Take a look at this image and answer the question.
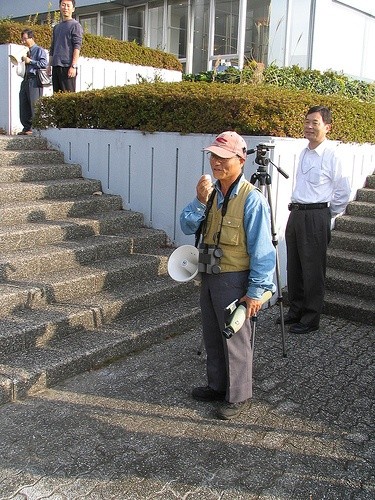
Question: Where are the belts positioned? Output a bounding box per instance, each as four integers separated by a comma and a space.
290, 203, 327, 210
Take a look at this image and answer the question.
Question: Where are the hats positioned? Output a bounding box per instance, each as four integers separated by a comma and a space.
17, 60, 26, 80
201, 131, 247, 160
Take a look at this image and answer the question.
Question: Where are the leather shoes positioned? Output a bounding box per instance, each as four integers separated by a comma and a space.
276, 315, 300, 323
289, 322, 319, 334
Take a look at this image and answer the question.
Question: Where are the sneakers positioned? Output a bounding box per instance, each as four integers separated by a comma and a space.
218, 399, 249, 419
192, 385, 227, 401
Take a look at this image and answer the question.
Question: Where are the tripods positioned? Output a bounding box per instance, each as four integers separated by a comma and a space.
245, 156, 289, 360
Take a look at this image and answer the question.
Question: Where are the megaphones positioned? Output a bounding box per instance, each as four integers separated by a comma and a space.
167, 244, 202, 287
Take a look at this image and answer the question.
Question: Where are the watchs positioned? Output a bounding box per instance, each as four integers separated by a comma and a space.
72, 64, 77, 69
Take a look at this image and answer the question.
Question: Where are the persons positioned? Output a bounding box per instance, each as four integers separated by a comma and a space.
180, 131, 276, 419
46, 0, 83, 129
10, 29, 51, 135
276, 108, 351, 334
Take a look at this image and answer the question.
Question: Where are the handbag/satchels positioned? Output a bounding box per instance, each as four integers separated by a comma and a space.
36, 47, 53, 87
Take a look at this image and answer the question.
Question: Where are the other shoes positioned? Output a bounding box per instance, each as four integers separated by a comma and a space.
18, 129, 33, 135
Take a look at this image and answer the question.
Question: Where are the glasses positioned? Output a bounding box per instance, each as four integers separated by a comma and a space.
206, 151, 219, 160
